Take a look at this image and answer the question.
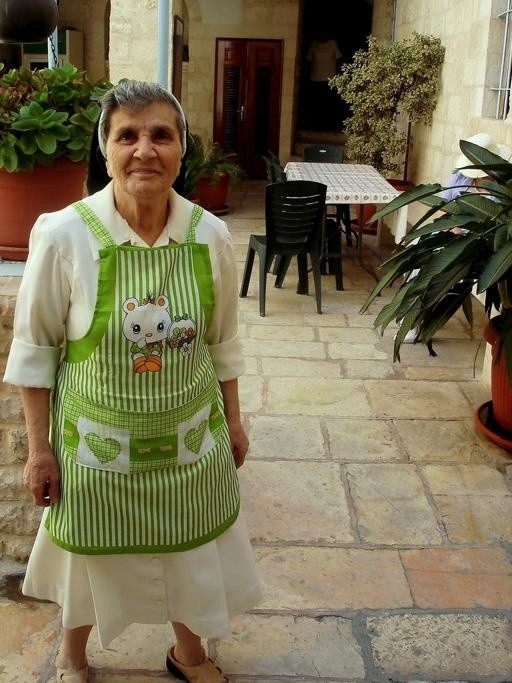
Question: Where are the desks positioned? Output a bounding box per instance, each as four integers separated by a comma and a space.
282, 161, 398, 294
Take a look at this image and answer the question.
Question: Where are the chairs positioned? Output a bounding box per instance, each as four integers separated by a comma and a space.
263, 145, 354, 292
239, 179, 333, 316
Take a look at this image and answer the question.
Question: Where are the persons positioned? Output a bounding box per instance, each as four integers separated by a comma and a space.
2, 77, 250, 682
390, 132, 511, 347
306, 34, 343, 83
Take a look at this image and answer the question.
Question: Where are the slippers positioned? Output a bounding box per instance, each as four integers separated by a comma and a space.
57, 664, 88, 683
166, 645, 229, 683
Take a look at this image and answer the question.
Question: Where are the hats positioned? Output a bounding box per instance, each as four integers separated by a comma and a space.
457, 133, 512, 178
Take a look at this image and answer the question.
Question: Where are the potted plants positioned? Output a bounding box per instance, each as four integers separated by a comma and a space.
0, 54, 127, 262
188, 135, 244, 218
366, 138, 512, 439
328, 29, 446, 236
